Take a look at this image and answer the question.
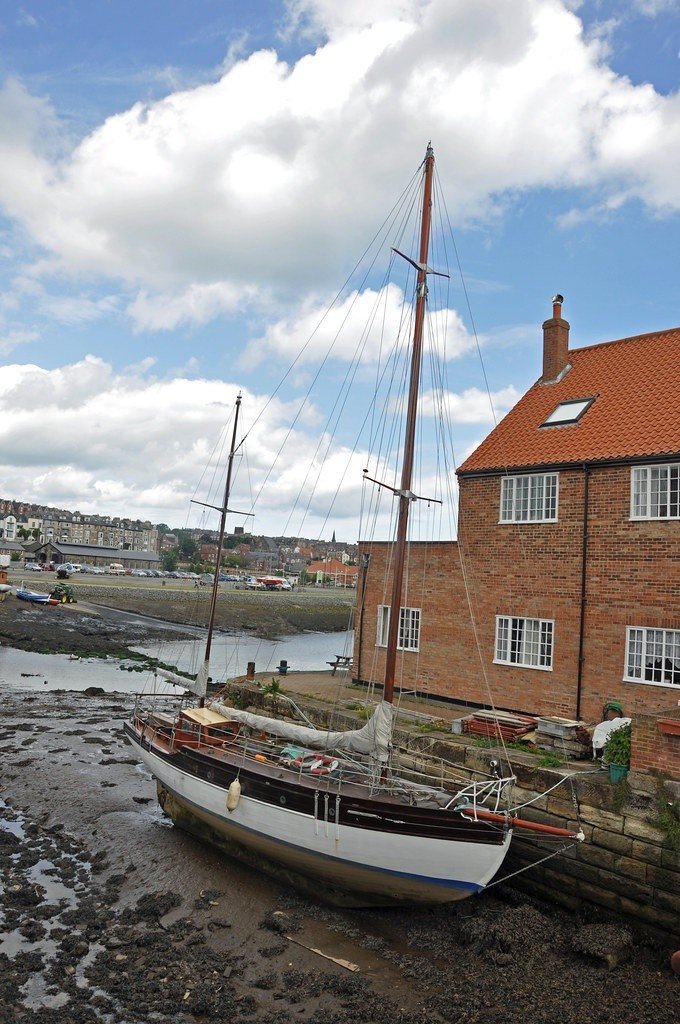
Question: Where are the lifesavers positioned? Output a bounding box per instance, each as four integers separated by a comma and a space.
310, 758, 339, 775
294, 752, 324, 767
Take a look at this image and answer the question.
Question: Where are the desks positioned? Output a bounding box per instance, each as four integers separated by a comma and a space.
327, 654, 354, 676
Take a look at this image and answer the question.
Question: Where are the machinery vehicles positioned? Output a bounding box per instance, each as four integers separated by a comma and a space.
50, 582, 77, 604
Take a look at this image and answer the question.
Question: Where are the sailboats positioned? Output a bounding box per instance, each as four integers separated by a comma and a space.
119, 140, 585, 903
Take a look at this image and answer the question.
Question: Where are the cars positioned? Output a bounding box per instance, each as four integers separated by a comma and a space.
23, 558, 292, 590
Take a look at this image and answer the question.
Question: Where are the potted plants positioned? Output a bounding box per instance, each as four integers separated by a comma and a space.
604, 722, 632, 787
